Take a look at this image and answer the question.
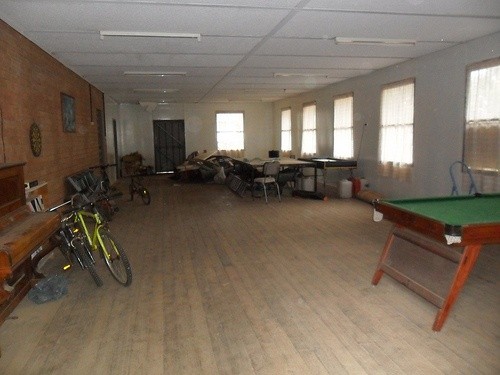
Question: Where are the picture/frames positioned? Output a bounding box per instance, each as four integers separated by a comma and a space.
60, 92, 77, 133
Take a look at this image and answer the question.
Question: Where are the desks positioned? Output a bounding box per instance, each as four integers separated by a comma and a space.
373, 193, 500, 331
236, 157, 316, 201
299, 158, 358, 201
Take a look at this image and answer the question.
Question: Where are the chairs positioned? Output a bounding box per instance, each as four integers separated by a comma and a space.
254, 161, 281, 202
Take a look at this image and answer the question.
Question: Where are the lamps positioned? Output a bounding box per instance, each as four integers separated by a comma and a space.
124, 72, 186, 75
100, 30, 203, 41
335, 37, 416, 47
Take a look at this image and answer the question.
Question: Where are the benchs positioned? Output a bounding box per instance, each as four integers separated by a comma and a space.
226, 173, 251, 198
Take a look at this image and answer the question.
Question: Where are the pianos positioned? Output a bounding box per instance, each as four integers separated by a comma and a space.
0, 162, 63, 329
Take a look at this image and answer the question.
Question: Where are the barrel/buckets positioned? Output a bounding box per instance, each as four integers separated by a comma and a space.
360, 177, 368, 191
348, 177, 360, 193
338, 179, 352, 199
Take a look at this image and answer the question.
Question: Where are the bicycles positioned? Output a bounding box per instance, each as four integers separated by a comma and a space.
128, 173, 151, 205
80, 162, 119, 221
49, 192, 133, 288
